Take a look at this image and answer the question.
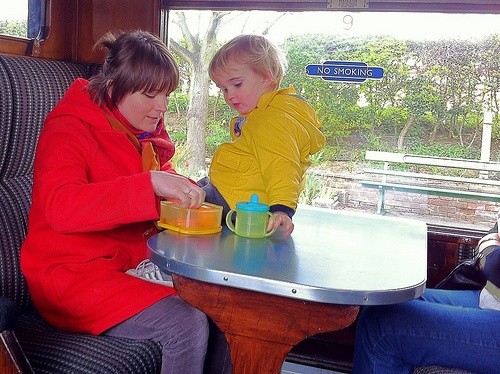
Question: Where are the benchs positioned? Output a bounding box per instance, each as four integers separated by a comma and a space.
360, 149, 500, 218
0, 53, 225, 374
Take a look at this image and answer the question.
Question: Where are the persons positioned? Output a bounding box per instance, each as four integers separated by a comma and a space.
351, 209, 500, 374
19, 29, 232, 374
196, 34, 325, 237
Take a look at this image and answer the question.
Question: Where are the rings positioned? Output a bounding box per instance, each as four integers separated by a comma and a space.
187, 187, 193, 196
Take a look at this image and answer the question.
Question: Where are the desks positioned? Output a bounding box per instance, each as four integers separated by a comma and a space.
147, 204, 427, 374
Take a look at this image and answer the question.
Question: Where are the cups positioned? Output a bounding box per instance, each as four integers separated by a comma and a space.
226, 194, 277, 239
